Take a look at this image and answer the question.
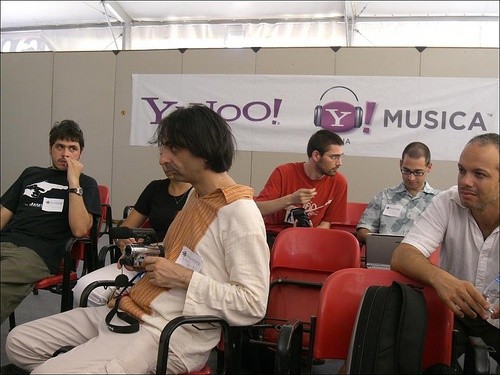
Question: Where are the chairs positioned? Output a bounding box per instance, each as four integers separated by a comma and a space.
80, 197, 453, 375
9, 184, 112, 330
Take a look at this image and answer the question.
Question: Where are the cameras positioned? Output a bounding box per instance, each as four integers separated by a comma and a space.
121, 244, 165, 267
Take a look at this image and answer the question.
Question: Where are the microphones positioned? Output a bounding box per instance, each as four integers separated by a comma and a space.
108, 226, 157, 240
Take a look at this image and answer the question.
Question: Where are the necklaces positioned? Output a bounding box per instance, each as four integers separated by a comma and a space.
172, 184, 188, 204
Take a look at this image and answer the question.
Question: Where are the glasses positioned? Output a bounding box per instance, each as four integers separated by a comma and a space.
401, 165, 427, 176
324, 154, 344, 161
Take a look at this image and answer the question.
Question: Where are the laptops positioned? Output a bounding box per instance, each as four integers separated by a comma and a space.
366, 234, 406, 267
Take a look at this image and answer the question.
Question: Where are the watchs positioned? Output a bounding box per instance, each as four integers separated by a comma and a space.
68, 187, 83, 196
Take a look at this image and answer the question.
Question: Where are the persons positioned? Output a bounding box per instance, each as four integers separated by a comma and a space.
356, 142, 439, 241
0, 120, 101, 327
5, 104, 270, 375
390, 132, 499, 375
70, 143, 194, 308
254, 130, 348, 230
